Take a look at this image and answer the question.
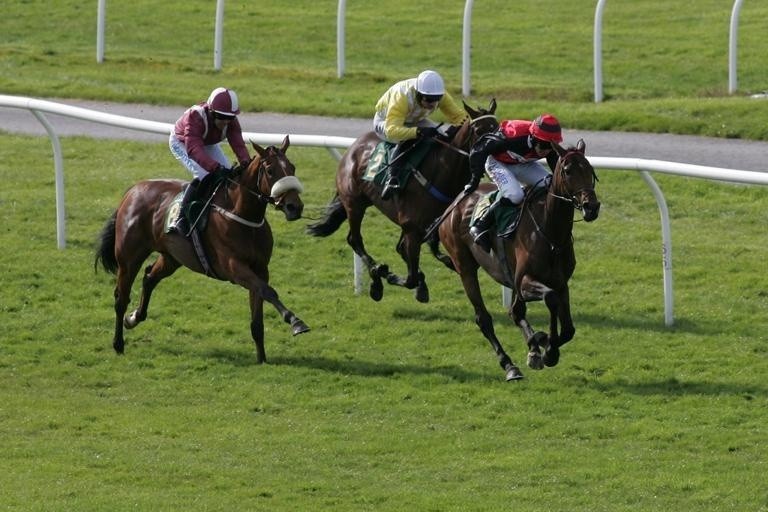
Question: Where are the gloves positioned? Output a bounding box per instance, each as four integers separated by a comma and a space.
217, 164, 228, 174
417, 127, 439, 138
463, 177, 478, 194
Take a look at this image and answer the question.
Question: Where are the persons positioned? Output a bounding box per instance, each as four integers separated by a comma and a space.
373, 70, 472, 143
169, 87, 251, 182
484, 114, 563, 209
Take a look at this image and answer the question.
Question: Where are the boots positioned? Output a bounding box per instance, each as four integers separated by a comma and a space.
163, 184, 195, 235
380, 147, 402, 201
469, 198, 519, 253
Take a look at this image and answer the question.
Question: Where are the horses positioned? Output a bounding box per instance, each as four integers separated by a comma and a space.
426, 138, 601, 382
306, 99, 499, 303
94, 134, 311, 365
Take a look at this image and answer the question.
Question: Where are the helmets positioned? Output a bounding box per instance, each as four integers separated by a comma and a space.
415, 70, 445, 95
207, 87, 241, 118
529, 115, 563, 144
